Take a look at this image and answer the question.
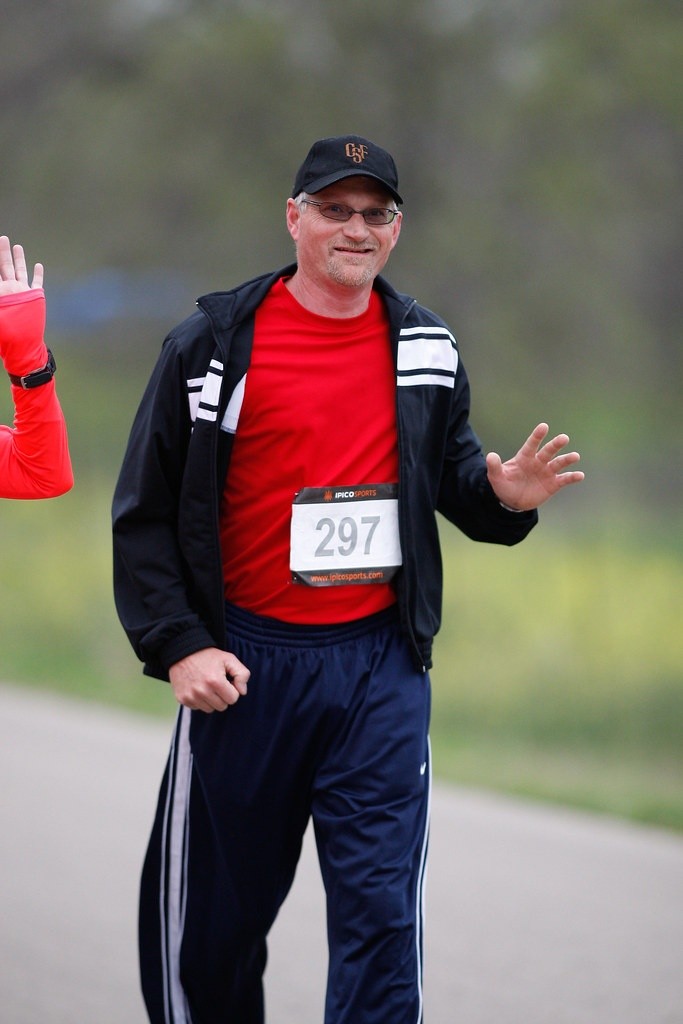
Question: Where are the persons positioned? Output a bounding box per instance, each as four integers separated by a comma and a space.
0, 236, 75, 504
113, 138, 585, 1024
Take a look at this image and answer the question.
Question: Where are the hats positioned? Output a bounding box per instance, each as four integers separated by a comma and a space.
292, 134, 403, 206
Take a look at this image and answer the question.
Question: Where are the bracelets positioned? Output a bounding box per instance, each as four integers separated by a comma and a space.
7, 347, 58, 388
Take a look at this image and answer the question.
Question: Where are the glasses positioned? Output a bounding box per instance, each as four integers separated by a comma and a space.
301, 198, 399, 225
